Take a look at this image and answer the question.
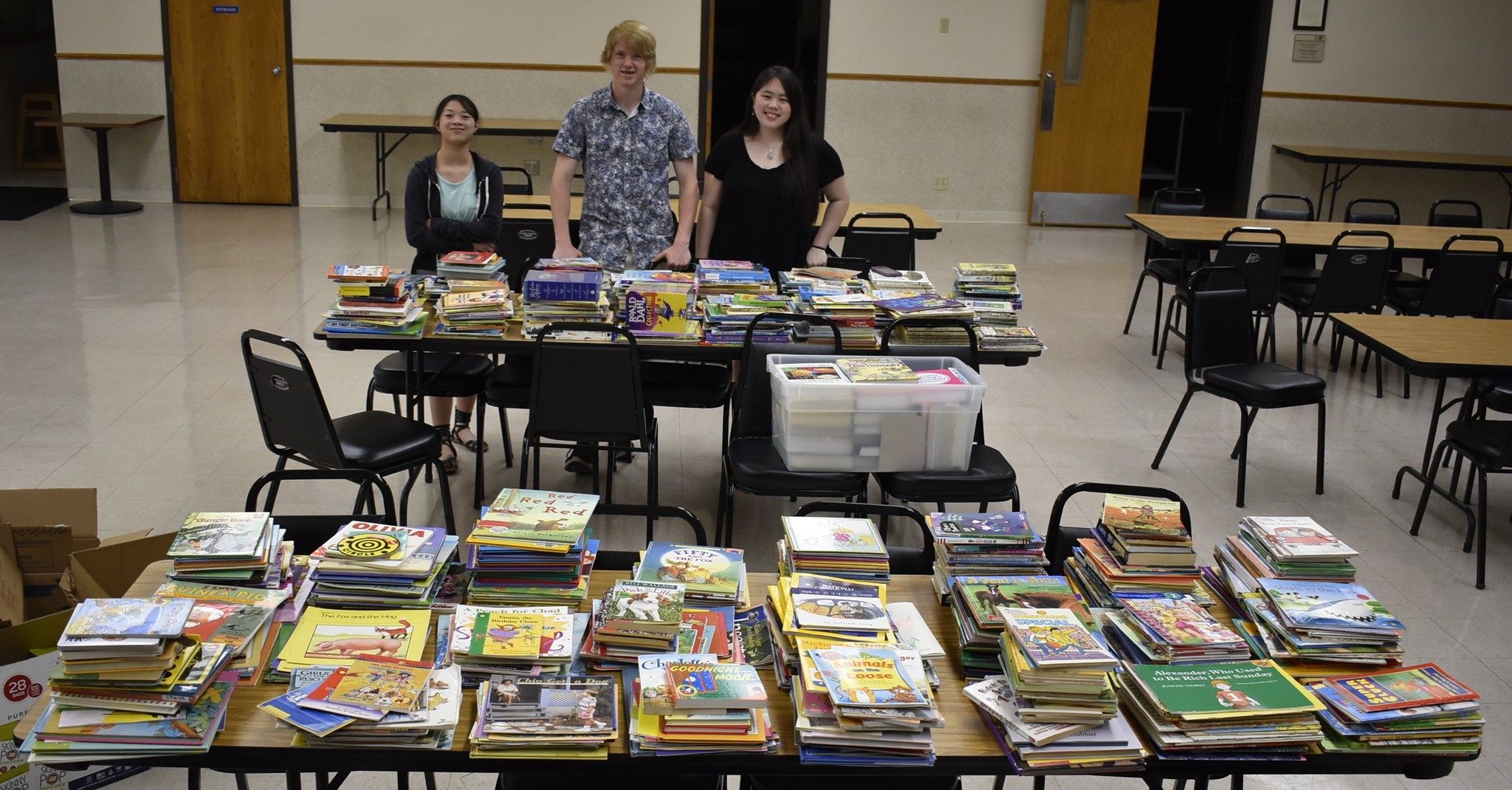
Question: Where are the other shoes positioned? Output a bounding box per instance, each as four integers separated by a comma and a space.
573, 728, 590, 732
598, 723, 607, 729
617, 442, 634, 464
564, 442, 596, 474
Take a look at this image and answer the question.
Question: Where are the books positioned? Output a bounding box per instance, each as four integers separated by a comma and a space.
778, 359, 975, 475
321, 251, 1041, 354
18, 490, 1486, 769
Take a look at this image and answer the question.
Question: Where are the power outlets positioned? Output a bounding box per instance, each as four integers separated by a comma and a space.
524, 161, 540, 176
527, 137, 543, 145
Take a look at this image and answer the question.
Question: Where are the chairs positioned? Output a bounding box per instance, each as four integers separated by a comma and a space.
187, 167, 1238, 790
1122, 194, 1512, 590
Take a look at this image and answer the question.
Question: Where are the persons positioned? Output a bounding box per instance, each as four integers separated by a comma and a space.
574, 689, 607, 732
497, 679, 518, 706
549, 20, 695, 473
405, 94, 504, 471
691, 66, 850, 388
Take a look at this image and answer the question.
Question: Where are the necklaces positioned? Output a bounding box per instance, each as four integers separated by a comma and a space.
756, 138, 783, 160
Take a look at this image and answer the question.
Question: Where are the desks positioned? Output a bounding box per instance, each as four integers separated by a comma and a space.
1272, 144, 1512, 223
502, 194, 943, 240
34, 112, 165, 215
14, 563, 1480, 790
312, 294, 1043, 366
320, 113, 564, 222
1125, 213, 1512, 318
1330, 313, 1512, 552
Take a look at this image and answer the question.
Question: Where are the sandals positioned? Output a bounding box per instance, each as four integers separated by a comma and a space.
436, 424, 458, 471
450, 406, 489, 453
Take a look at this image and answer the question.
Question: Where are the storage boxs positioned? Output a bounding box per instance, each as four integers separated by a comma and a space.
0, 488, 181, 790
766, 353, 987, 472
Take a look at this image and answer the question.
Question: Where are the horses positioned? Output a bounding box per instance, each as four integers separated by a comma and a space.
975, 584, 1010, 616
1011, 593, 1091, 623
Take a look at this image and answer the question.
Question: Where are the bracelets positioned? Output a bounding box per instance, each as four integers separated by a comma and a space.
812, 245, 825, 252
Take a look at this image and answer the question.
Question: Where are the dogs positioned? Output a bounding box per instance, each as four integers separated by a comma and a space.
614, 591, 671, 621
